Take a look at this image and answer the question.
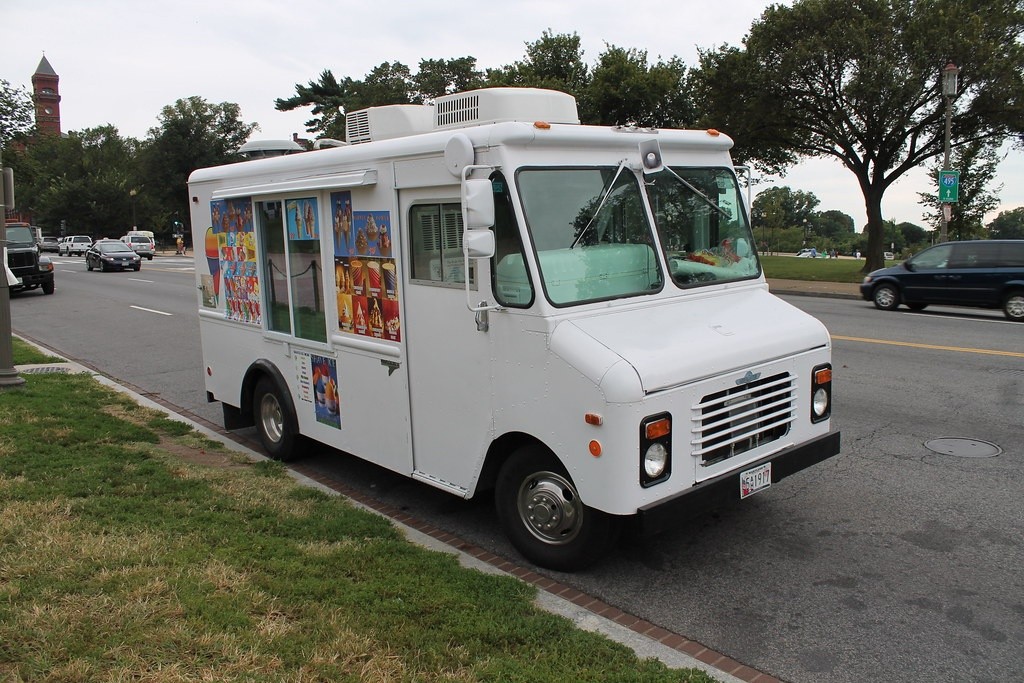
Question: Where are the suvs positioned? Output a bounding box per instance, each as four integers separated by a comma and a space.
37, 237, 60, 253
57, 236, 92, 257
4, 222, 54, 298
120, 235, 155, 260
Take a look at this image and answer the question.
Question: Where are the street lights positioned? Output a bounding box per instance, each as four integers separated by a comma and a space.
130, 188, 137, 231
940, 60, 957, 241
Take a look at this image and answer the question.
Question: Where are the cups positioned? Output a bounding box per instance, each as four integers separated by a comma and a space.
351, 260, 363, 296
316, 374, 337, 415
367, 261, 381, 298
381, 263, 396, 299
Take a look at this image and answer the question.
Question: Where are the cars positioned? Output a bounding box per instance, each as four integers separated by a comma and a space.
85, 237, 126, 257
86, 244, 141, 273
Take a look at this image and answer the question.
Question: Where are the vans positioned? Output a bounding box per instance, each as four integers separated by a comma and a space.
128, 231, 156, 247
859, 240, 1024, 322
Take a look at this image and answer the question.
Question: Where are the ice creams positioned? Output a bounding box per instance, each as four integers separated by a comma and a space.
212, 201, 253, 232
355, 214, 391, 257
313, 363, 338, 416
333, 199, 351, 250
295, 200, 315, 238
336, 269, 399, 340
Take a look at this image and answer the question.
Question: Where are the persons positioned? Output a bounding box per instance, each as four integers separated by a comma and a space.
811, 247, 816, 258
177, 236, 186, 254
822, 249, 838, 259
856, 250, 861, 259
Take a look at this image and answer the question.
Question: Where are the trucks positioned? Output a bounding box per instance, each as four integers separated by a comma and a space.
186, 85, 841, 572
